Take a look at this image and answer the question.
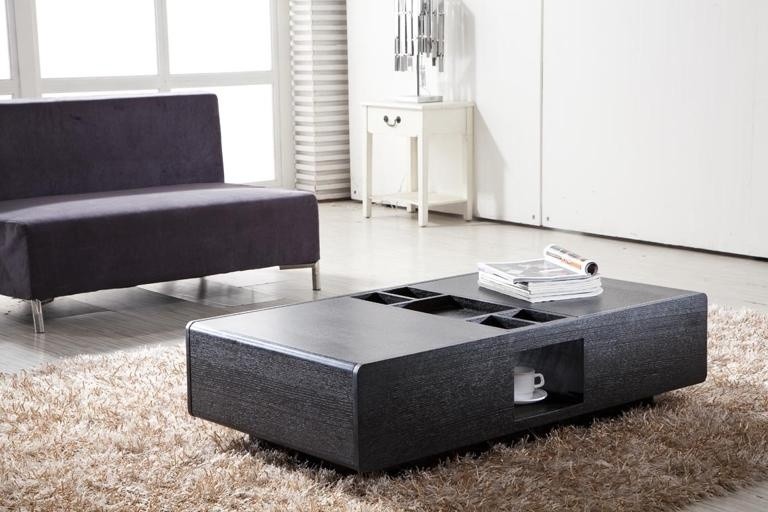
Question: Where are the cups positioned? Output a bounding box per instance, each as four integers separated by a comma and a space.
514, 366, 545, 396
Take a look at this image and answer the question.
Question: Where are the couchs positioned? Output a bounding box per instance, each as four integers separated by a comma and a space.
0, 94, 320, 333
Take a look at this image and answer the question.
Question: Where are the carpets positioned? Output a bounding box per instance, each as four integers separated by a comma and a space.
0, 305, 768, 512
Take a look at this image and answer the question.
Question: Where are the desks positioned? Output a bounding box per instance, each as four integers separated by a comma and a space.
185, 272, 708, 473
363, 101, 476, 227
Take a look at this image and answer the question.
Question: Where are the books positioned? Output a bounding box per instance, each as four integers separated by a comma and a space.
476, 242, 604, 303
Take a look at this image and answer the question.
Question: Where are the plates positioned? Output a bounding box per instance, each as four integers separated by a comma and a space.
514, 389, 548, 405
